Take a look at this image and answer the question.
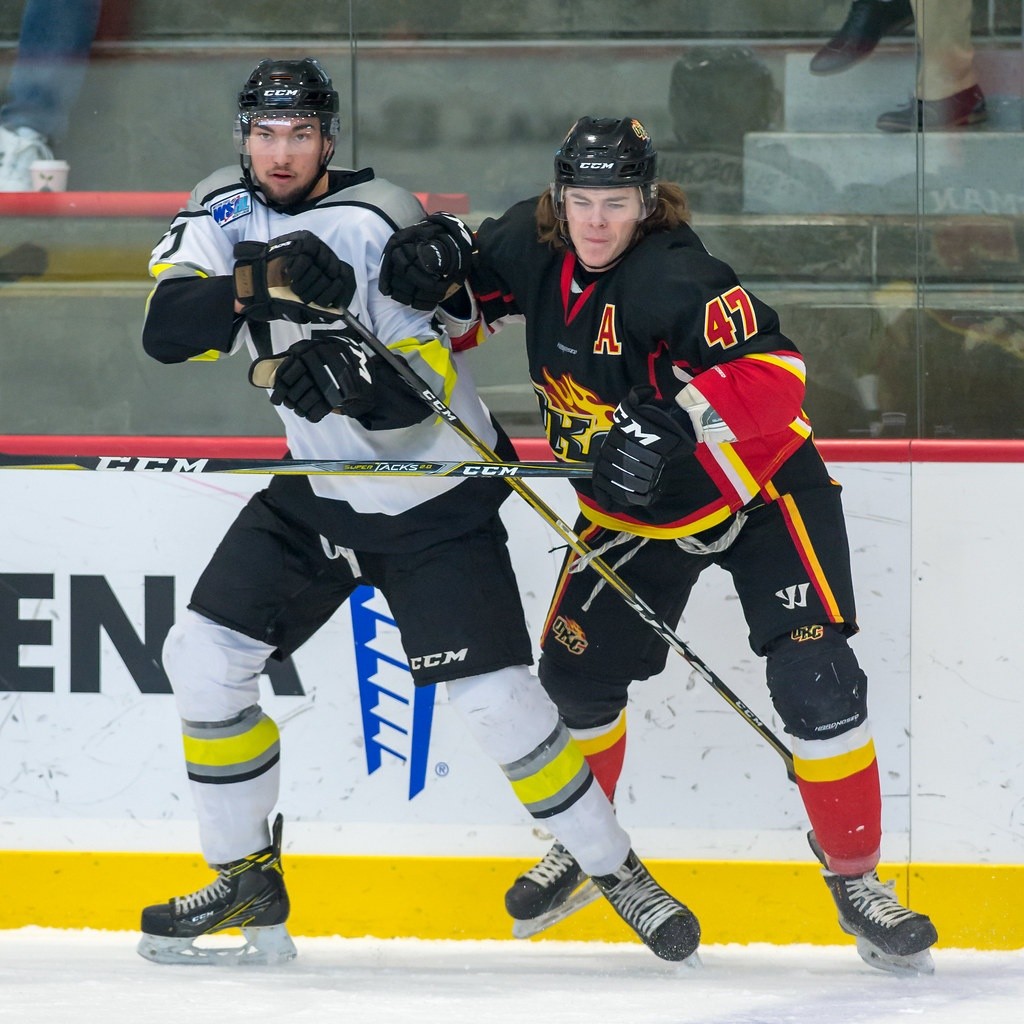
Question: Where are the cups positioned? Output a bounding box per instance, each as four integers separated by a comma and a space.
30, 159, 70, 192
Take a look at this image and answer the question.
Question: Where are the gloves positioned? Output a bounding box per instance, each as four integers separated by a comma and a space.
592, 385, 698, 513
249, 336, 380, 424
378, 211, 478, 312
232, 230, 357, 325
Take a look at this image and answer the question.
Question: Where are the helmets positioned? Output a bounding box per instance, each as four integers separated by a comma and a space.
555, 116, 657, 218
668, 45, 774, 143
238, 58, 340, 139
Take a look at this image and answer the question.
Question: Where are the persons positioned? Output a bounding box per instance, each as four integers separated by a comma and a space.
0, 0, 103, 193
140, 56, 701, 963
378, 117, 938, 957
651, 45, 1024, 440
810, 0, 987, 133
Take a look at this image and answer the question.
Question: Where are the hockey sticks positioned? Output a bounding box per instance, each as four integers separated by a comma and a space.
341, 308, 800, 787
1, 453, 600, 479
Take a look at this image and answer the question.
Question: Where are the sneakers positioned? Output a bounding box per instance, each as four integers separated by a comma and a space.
503, 783, 617, 939
136, 813, 298, 969
807, 829, 938, 977
0, 126, 53, 192
590, 848, 704, 972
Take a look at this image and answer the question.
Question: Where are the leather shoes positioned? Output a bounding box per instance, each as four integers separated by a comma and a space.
809, 0, 916, 76
875, 82, 987, 132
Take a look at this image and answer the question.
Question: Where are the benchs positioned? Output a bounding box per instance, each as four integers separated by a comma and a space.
1, 1, 1024, 471
